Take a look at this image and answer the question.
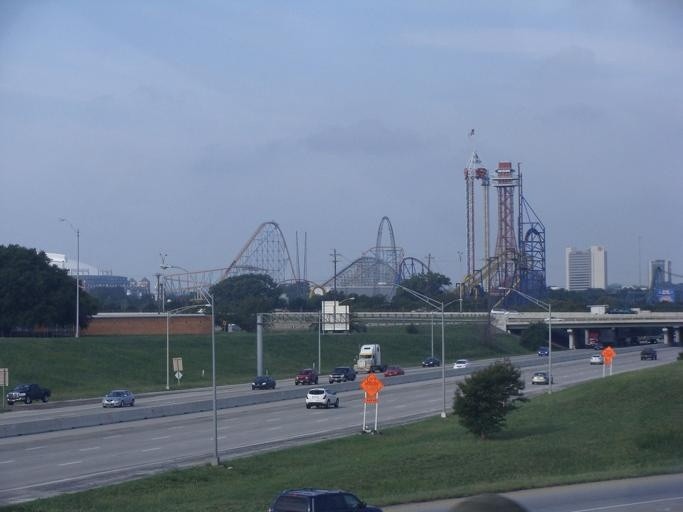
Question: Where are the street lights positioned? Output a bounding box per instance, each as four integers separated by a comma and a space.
498, 286, 552, 395
318, 297, 356, 374
378, 281, 464, 418
59, 216, 80, 337
159, 250, 220, 466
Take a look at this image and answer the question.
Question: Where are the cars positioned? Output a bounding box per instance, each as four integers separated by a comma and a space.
453, 359, 469, 369
590, 355, 603, 365
269, 487, 382, 512
102, 389, 136, 408
605, 307, 638, 314
306, 388, 339, 409
295, 368, 318, 385
640, 348, 657, 360
538, 347, 549, 356
532, 372, 553, 385
251, 376, 276, 390
329, 367, 356, 384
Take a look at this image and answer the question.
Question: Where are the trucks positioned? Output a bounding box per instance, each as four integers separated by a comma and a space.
353, 343, 387, 373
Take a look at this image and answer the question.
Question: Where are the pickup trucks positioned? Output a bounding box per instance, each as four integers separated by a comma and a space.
6, 383, 51, 405
